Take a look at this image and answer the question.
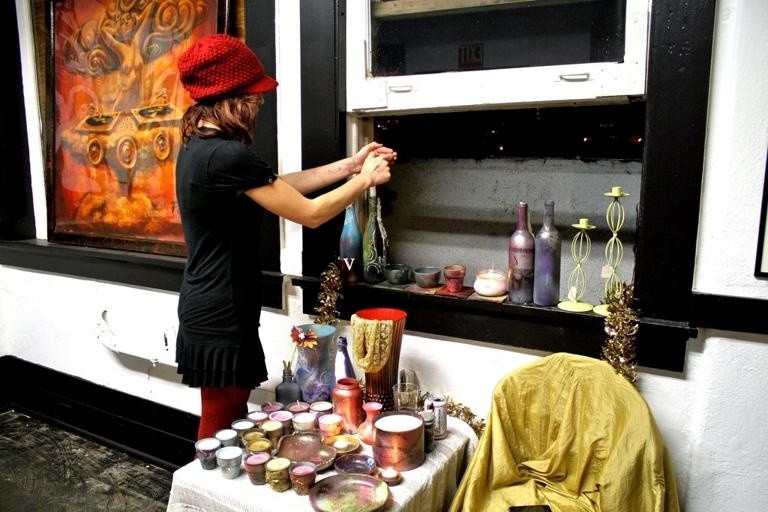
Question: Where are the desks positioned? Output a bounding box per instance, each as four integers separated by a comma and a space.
163, 416, 478, 512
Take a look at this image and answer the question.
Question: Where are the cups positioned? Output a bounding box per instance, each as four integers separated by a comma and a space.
195, 439, 220, 469
310, 402, 332, 426
444, 264, 466, 294
265, 458, 291, 493
260, 421, 284, 444
215, 428, 238, 446
393, 383, 417, 415
269, 410, 293, 433
244, 452, 271, 485
371, 411, 425, 472
247, 438, 272, 454
318, 414, 343, 436
232, 419, 255, 436
293, 412, 314, 432
247, 411, 268, 425
242, 429, 265, 445
216, 446, 241, 479
261, 401, 285, 412
288, 401, 309, 412
289, 462, 316, 497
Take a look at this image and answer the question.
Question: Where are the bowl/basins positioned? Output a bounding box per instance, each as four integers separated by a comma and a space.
413, 266, 441, 288
271, 429, 338, 471
335, 454, 377, 473
473, 270, 507, 296
323, 435, 361, 453
385, 263, 412, 285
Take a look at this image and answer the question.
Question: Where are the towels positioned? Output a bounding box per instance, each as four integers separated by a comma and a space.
351, 314, 392, 374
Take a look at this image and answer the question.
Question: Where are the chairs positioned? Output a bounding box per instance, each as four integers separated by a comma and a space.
451, 353, 680, 512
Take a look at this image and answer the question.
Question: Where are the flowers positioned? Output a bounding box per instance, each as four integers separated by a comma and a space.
291, 326, 317, 348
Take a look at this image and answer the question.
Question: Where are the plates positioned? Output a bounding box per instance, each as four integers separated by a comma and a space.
310, 474, 389, 512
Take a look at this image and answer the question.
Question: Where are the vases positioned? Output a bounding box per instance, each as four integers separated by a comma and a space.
355, 307, 408, 410
296, 323, 337, 402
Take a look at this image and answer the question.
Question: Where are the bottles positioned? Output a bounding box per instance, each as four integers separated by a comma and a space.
274, 369, 301, 403
340, 202, 363, 284
332, 378, 362, 429
421, 412, 437, 453
534, 200, 561, 307
362, 195, 387, 283
509, 202, 534, 303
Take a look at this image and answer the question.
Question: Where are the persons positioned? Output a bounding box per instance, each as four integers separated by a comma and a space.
174, 33, 398, 441
99, 1, 158, 113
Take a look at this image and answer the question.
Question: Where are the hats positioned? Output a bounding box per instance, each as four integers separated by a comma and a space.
177, 33, 279, 102
